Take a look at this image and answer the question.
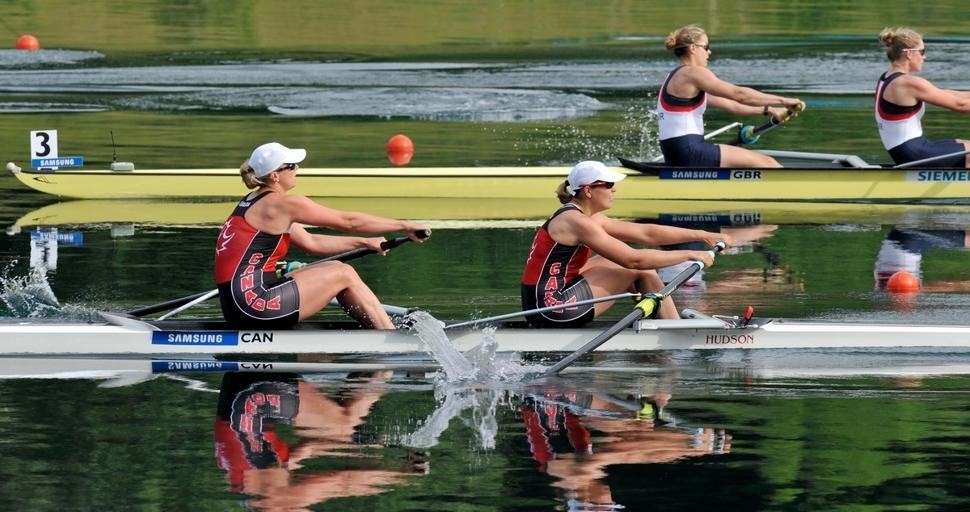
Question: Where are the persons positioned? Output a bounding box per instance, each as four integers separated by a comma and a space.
658, 26, 806, 168
214, 368, 429, 508
664, 216, 806, 312
216, 143, 432, 329
526, 351, 735, 511
872, 227, 970, 294
876, 26, 970, 169
517, 159, 734, 316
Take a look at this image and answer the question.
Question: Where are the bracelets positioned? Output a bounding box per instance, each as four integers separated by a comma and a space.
762, 104, 773, 115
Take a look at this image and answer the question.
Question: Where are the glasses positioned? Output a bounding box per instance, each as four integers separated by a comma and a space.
695, 43, 710, 52
581, 182, 615, 190
276, 164, 297, 172
902, 47, 927, 56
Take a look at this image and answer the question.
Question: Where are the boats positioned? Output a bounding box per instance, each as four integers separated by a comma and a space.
2, 351, 970, 395
3, 155, 970, 201
2, 308, 970, 358
11, 194, 967, 240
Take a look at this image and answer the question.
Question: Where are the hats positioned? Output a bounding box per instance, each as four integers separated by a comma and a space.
247, 142, 308, 179
566, 160, 627, 190
